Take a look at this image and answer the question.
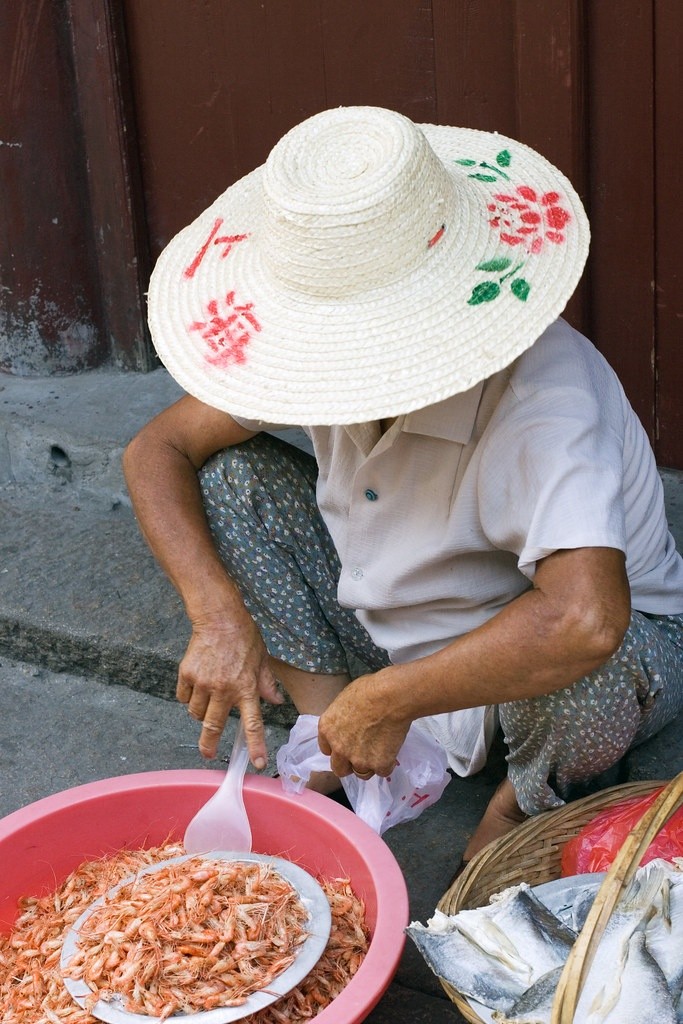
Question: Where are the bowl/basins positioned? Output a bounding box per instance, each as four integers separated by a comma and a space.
0, 767, 409, 1024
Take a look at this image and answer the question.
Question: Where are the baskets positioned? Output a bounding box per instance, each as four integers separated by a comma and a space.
429, 771, 683, 1024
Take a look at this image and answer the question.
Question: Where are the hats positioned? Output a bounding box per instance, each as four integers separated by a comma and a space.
146, 104, 590, 426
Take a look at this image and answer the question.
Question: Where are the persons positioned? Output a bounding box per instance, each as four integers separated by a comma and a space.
122, 105, 683, 892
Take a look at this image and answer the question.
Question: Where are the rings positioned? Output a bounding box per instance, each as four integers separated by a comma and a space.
353, 769, 372, 776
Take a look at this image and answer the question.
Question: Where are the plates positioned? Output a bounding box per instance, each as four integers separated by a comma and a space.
60, 851, 332, 1024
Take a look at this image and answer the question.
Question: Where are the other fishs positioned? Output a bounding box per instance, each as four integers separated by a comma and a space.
403, 856, 683, 1024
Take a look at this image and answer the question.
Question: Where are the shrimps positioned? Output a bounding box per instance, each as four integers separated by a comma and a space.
0, 828, 369, 1024
